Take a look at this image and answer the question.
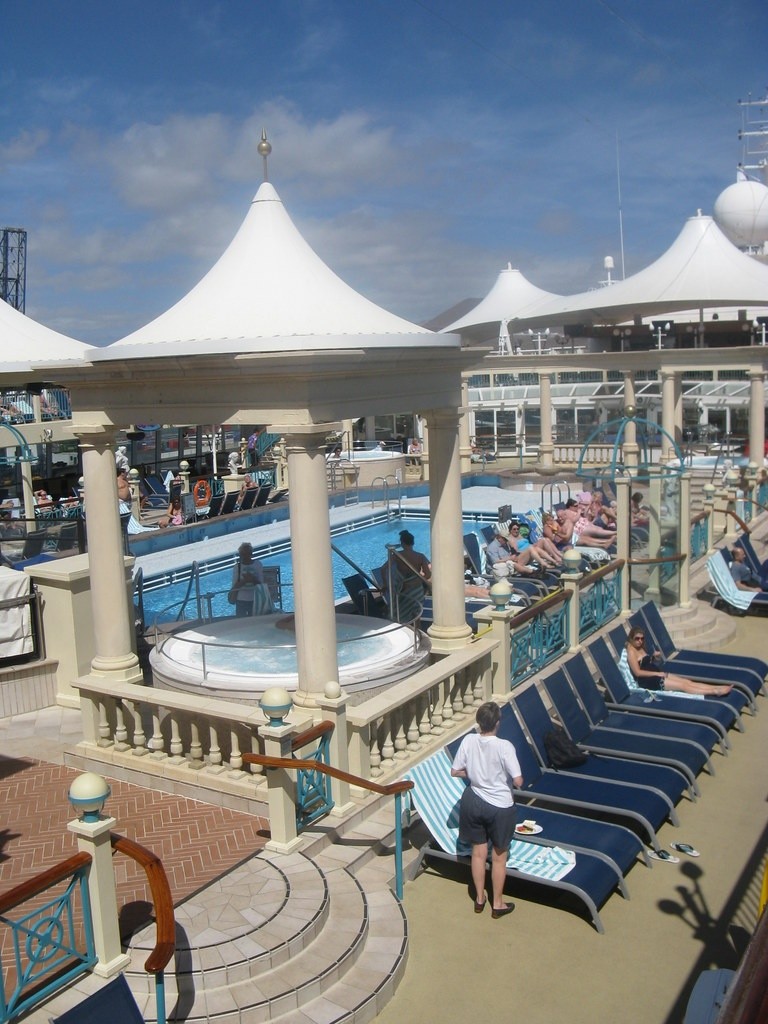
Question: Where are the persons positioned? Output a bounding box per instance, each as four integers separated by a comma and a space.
116, 446, 130, 473
34, 490, 79, 513
0, 500, 25, 528
486, 528, 561, 577
471, 441, 489, 458
564, 490, 617, 549
507, 522, 563, 562
140, 491, 148, 509
166, 499, 182, 525
232, 543, 264, 617
334, 447, 341, 457
410, 438, 422, 465
0, 391, 59, 417
731, 547, 768, 592
542, 509, 617, 548
117, 467, 132, 509
248, 430, 261, 466
624, 626, 734, 698
228, 452, 240, 474
503, 541, 562, 569
238, 475, 259, 504
380, 530, 514, 629
631, 492, 674, 526
450, 702, 523, 919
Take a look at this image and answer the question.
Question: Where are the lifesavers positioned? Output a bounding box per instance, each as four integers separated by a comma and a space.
194, 480, 211, 506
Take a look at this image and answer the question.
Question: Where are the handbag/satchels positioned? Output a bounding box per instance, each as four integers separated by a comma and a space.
227, 589, 238, 604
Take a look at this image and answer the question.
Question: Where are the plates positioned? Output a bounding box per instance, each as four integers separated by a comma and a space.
514, 823, 544, 835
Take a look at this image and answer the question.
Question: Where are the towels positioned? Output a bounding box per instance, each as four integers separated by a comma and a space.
617, 647, 703, 700
162, 470, 175, 493
531, 508, 611, 562
576, 491, 592, 505
704, 551, 759, 611
469, 531, 488, 586
398, 746, 577, 882
251, 583, 286, 616
493, 519, 515, 535
119, 498, 160, 534
386, 569, 425, 624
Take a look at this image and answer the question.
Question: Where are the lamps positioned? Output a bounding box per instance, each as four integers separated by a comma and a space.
563, 550, 582, 574
748, 462, 758, 475
518, 404, 522, 415
68, 772, 111, 823
273, 446, 281, 456
129, 469, 138, 481
727, 473, 738, 489
180, 461, 189, 472
488, 582, 512, 612
78, 477, 84, 488
702, 484, 716, 500
258, 687, 295, 728
698, 401, 703, 413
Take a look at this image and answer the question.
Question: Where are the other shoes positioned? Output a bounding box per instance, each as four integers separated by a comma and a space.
475, 896, 487, 913
538, 565, 546, 578
492, 903, 515, 920
529, 568, 542, 580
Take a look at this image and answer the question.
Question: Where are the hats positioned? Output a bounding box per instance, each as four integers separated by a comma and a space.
498, 529, 511, 541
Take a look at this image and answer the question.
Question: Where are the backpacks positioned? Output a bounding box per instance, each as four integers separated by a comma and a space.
544, 727, 588, 773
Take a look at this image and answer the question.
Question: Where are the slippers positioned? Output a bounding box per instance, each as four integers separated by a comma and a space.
669, 842, 700, 856
648, 850, 680, 864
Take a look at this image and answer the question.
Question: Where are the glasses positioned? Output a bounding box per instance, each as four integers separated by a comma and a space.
571, 505, 579, 508
633, 636, 644, 642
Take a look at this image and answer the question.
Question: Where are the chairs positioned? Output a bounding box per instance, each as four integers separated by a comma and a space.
399, 600, 768, 935
0, 471, 289, 566
0, 390, 71, 425
705, 534, 768, 610
471, 454, 497, 464
407, 438, 419, 466
341, 482, 650, 635
